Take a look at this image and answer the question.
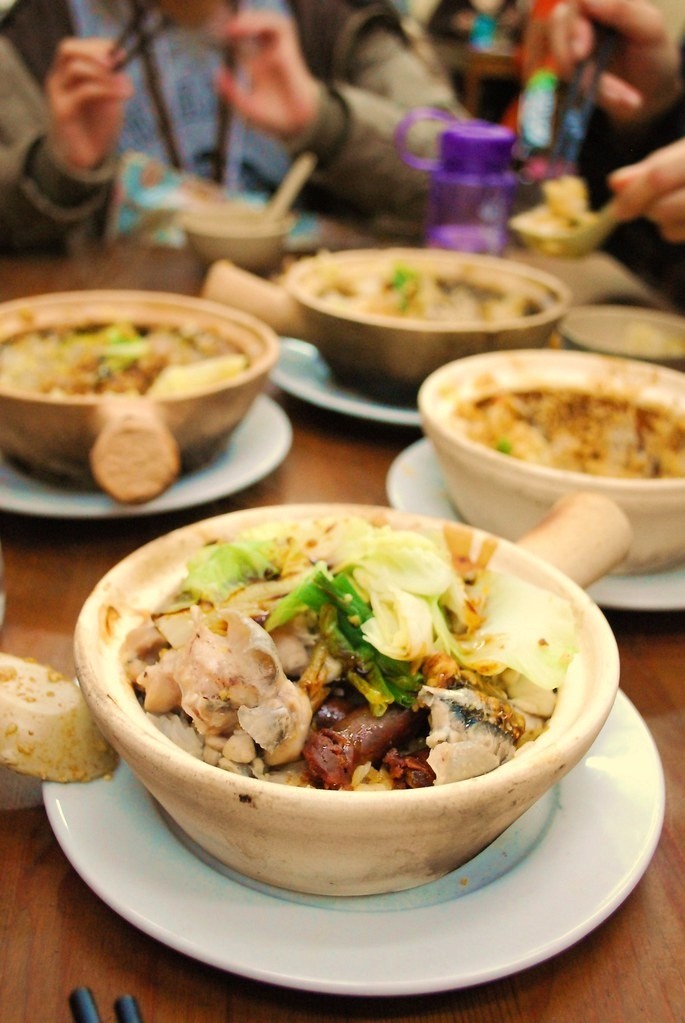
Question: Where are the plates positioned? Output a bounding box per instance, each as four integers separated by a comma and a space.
267, 335, 420, 425
0, 390, 291, 515
556, 302, 685, 362
385, 439, 685, 611
42, 672, 666, 996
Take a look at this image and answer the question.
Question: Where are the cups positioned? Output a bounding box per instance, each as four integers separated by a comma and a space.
393, 106, 513, 257
470, 16, 496, 52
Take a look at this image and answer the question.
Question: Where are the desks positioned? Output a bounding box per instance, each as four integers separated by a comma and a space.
0, 231, 684, 1023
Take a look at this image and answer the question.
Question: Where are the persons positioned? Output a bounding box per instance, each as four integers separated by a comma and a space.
427, 0, 559, 134
0, 0, 475, 251
545, 0, 685, 243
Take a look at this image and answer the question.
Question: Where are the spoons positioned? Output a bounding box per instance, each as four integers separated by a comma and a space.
507, 195, 621, 240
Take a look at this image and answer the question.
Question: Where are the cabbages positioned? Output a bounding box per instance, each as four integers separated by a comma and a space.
39, 315, 259, 401
184, 511, 577, 698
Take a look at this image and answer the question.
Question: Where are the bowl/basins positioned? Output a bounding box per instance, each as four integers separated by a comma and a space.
71, 493, 636, 890
0, 290, 280, 505
183, 211, 297, 269
204, 250, 574, 406
418, 349, 685, 574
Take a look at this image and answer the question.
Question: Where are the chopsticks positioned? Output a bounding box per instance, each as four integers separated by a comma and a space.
527, 41, 611, 180
68, 987, 143, 1023
105, 0, 167, 75
